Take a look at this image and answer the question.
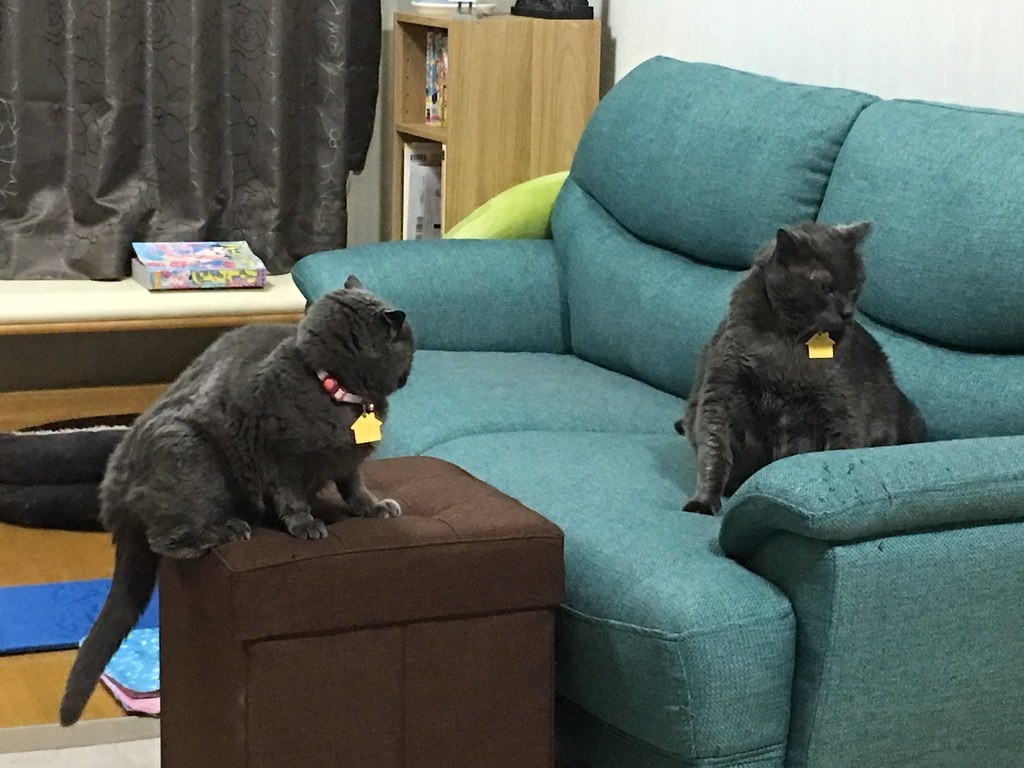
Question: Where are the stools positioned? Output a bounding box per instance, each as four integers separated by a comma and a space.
159, 456, 568, 768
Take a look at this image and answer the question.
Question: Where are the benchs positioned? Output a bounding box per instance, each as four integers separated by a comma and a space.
0, 271, 307, 393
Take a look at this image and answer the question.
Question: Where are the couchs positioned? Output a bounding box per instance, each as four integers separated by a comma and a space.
290, 55, 1024, 768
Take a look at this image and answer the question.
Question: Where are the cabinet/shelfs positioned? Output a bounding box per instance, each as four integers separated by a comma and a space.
389, 12, 602, 241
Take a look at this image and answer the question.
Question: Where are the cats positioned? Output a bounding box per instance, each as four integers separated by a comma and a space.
58, 275, 414, 728
673, 222, 926, 516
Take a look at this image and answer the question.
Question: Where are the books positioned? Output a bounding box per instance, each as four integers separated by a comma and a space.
401, 30, 448, 245
130, 239, 267, 290
